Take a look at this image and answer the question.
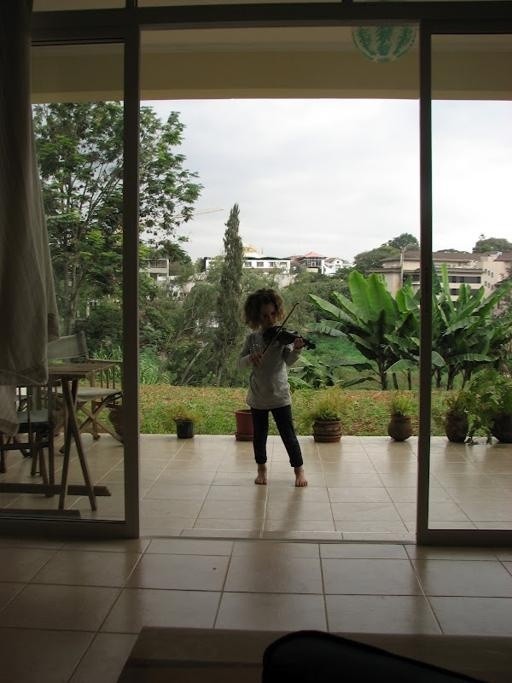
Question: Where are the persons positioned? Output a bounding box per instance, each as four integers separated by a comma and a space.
235, 287, 310, 487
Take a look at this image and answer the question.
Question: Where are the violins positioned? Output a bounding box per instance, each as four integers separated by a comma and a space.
263, 325, 316, 350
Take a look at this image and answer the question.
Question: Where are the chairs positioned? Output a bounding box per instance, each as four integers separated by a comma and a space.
0, 331, 123, 517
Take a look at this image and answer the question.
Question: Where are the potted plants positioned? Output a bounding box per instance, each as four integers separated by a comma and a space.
234, 378, 312, 441
301, 388, 353, 443
433, 368, 512, 446
165, 403, 204, 439
388, 397, 417, 441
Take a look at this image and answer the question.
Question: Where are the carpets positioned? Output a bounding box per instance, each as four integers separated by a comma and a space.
116, 627, 512, 683
